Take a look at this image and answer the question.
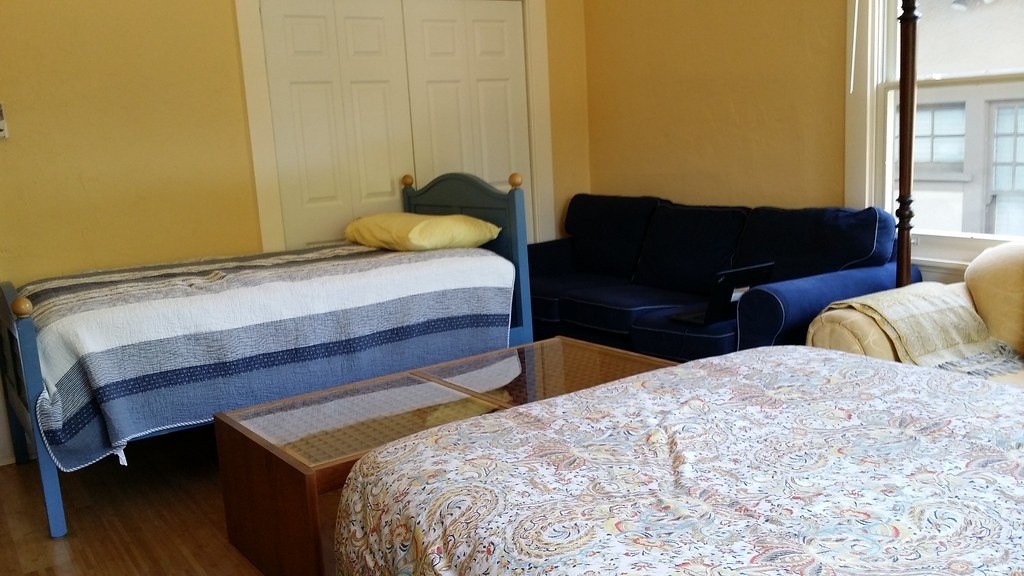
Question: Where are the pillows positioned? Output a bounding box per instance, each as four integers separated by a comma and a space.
344, 212, 503, 251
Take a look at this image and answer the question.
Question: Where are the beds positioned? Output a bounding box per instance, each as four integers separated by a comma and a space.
0, 170, 539, 539
339, 346, 1024, 576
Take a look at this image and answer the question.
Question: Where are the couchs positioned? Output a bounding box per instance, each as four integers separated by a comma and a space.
807, 235, 1024, 387
520, 186, 916, 350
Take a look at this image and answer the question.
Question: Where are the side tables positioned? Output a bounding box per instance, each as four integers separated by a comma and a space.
209, 331, 683, 573
